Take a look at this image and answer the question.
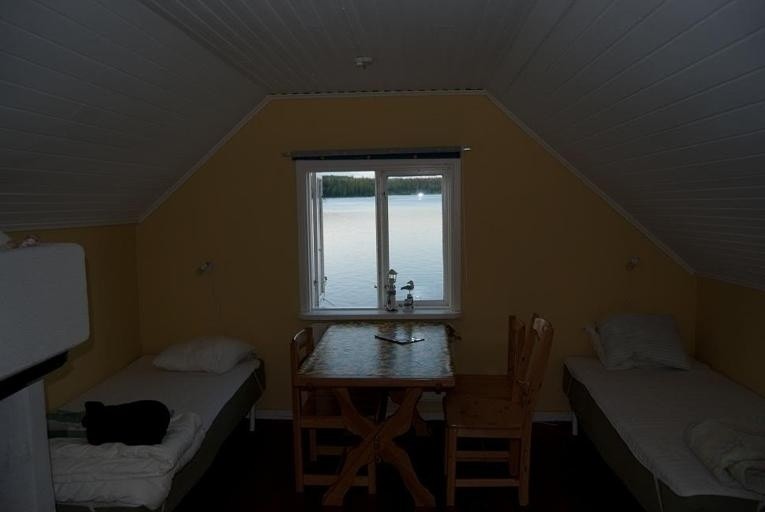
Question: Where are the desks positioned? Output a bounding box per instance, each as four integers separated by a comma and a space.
291, 320, 457, 506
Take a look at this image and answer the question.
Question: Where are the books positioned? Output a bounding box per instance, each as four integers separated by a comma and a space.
374, 329, 425, 344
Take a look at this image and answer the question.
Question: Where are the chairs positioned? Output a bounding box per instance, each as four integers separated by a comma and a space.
290, 327, 379, 495
443, 315, 529, 402
442, 316, 553, 507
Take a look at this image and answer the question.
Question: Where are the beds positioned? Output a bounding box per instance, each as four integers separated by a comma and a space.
47, 354, 265, 512
562, 356, 765, 512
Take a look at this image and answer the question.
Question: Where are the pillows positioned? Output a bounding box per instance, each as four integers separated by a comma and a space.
152, 338, 256, 375
592, 318, 689, 370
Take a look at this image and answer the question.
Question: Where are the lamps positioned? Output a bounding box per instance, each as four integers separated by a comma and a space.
625, 256, 641, 272
